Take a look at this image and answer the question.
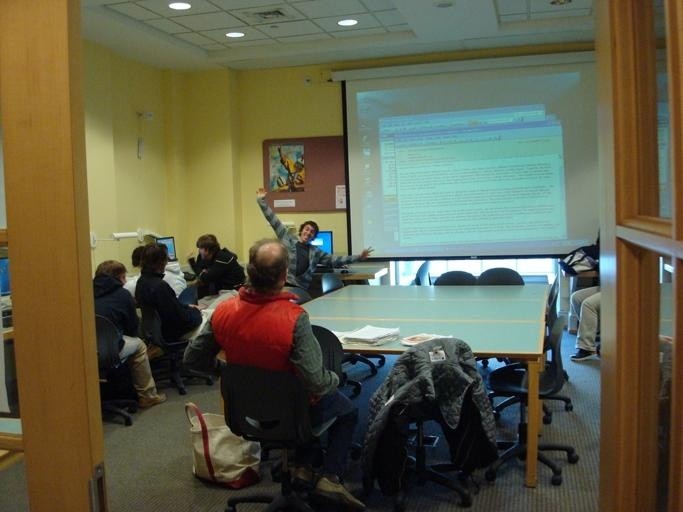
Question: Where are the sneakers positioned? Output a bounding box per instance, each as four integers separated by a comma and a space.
570, 348, 597, 361
139, 392, 167, 408
315, 478, 366, 511
290, 468, 313, 490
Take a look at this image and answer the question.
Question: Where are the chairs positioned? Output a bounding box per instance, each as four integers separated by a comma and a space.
365, 338, 473, 503
221, 369, 352, 510
92, 259, 565, 425
490, 310, 568, 427
486, 316, 567, 486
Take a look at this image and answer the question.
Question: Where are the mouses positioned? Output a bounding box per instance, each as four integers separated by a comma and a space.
341, 270, 349, 273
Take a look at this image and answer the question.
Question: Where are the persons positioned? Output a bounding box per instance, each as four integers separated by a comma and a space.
181, 237, 368, 510
134, 241, 209, 340
255, 186, 375, 289
92, 259, 169, 408
569, 284, 602, 361
176, 233, 248, 381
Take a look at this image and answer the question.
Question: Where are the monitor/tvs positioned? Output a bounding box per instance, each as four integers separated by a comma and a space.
157, 236, 177, 260
306, 230, 334, 257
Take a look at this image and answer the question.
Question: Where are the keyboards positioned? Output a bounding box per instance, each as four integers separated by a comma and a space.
315, 266, 334, 272
183, 272, 195, 281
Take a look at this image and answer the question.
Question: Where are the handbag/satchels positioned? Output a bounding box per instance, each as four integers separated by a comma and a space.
558, 245, 599, 276
185, 402, 262, 489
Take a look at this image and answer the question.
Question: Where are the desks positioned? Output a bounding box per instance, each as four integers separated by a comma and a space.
300, 283, 551, 486
